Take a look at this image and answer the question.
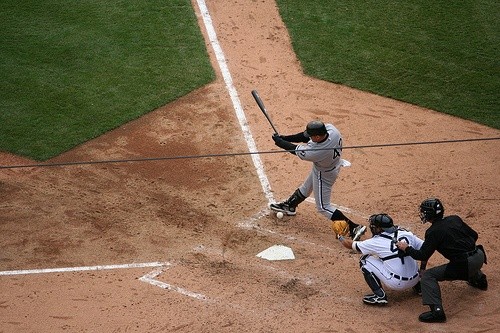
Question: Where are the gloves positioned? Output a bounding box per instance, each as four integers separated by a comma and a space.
271, 132, 285, 141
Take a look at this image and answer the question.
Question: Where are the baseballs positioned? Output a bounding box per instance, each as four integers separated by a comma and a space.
276, 212, 284, 218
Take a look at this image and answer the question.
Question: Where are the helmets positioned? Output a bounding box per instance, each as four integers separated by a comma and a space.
303, 121, 325, 137
368, 214, 393, 228
417, 198, 443, 223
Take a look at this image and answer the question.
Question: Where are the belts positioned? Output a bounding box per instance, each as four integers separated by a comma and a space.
454, 246, 479, 259
390, 272, 418, 281
325, 166, 336, 171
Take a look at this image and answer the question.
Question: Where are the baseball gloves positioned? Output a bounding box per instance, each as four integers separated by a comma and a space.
331, 220, 350, 239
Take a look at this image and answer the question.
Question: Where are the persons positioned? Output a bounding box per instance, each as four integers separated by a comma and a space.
395, 197, 488, 322
338, 212, 424, 306
269, 120, 367, 240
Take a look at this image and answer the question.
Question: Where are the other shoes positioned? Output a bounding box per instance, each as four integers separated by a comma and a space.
465, 274, 487, 291
270, 201, 296, 215
414, 284, 422, 295
348, 224, 367, 241
419, 306, 446, 322
362, 293, 388, 305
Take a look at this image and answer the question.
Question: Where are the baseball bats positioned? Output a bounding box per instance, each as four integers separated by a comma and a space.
251, 90, 281, 136
